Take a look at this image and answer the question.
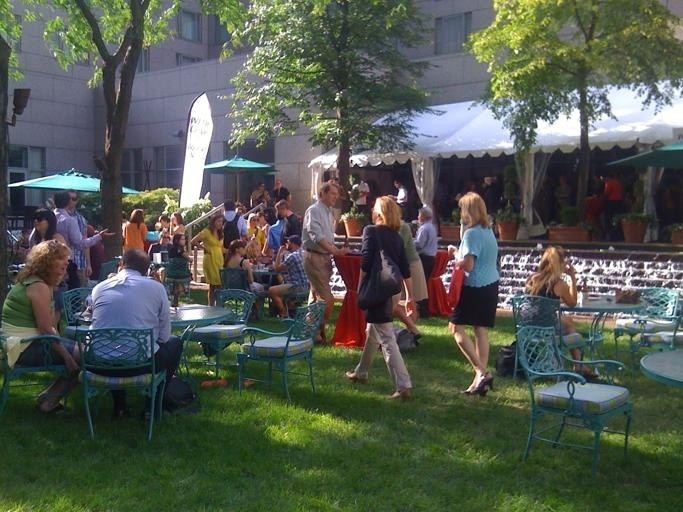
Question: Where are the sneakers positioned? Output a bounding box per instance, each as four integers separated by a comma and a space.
387, 389, 411, 398
346, 371, 368, 381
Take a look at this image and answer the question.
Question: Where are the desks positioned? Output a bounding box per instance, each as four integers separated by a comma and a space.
640, 348, 683, 389
557, 298, 647, 376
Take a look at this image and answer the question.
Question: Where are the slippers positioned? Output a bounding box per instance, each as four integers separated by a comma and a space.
36, 402, 70, 413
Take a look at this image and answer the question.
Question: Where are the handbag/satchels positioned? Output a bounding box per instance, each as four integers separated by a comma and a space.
447, 269, 464, 308
158, 376, 195, 411
357, 257, 402, 310
496, 341, 524, 379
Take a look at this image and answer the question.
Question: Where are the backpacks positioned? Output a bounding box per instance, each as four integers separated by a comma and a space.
222, 215, 240, 248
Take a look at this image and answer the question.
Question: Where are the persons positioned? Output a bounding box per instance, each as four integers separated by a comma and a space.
29, 187, 116, 318
301, 179, 352, 344
525, 245, 600, 378
392, 221, 428, 340
1, 239, 85, 415
413, 207, 438, 319
344, 196, 413, 398
191, 182, 309, 318
328, 169, 625, 243
85, 248, 183, 422
448, 191, 499, 397
122, 210, 194, 306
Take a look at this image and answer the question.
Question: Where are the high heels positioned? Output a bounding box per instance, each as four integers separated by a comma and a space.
463, 371, 494, 394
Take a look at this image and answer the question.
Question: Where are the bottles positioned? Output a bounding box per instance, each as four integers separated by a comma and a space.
581, 278, 589, 306
171, 279, 178, 307
343, 234, 349, 249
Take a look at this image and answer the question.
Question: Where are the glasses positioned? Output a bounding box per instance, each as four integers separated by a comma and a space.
69, 197, 77, 201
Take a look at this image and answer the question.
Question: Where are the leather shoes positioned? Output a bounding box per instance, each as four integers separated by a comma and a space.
141, 408, 151, 420
113, 407, 127, 423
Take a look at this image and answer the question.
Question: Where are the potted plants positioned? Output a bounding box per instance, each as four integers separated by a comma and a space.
494, 165, 522, 241
337, 186, 367, 238
437, 206, 460, 241
613, 176, 654, 245
663, 222, 683, 243
546, 205, 597, 244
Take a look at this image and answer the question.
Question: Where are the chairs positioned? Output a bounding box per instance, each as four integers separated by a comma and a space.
509, 296, 586, 387
612, 287, 682, 353
516, 325, 636, 478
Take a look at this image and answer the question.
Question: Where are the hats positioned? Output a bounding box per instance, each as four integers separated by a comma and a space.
230, 239, 246, 248
284, 235, 301, 245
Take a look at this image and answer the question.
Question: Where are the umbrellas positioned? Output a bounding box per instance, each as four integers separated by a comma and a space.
203, 155, 272, 201
607, 141, 683, 169
7, 168, 140, 194
